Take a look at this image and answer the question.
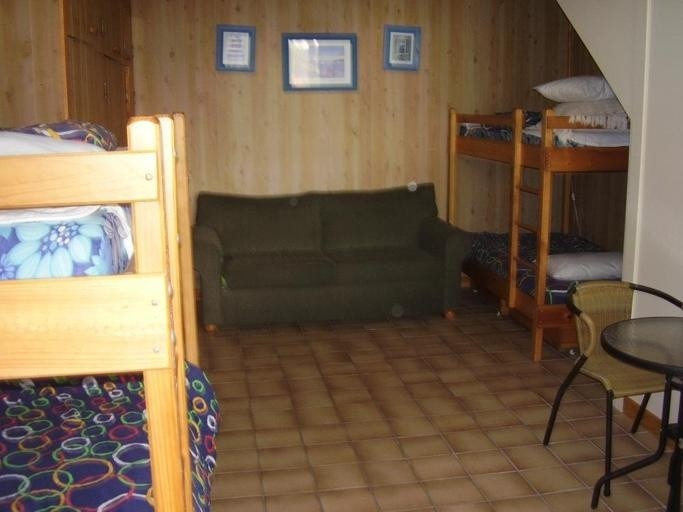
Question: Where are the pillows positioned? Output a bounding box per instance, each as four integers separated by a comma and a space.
533, 251, 636, 281
532, 77, 613, 106
0, 126, 108, 226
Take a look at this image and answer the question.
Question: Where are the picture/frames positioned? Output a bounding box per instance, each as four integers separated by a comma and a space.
216, 25, 255, 73
383, 25, 421, 71
282, 33, 358, 91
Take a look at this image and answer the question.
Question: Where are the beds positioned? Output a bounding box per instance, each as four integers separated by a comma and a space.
1, 114, 197, 512
447, 108, 629, 365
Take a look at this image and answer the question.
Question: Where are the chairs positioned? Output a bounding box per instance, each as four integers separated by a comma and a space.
544, 277, 682, 511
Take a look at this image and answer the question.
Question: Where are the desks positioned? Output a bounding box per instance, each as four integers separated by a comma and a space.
601, 315, 682, 511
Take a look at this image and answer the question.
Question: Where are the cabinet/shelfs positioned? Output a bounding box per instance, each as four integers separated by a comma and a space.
0, 0, 137, 149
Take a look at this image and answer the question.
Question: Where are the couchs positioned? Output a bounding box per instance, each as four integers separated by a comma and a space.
195, 183, 473, 328
